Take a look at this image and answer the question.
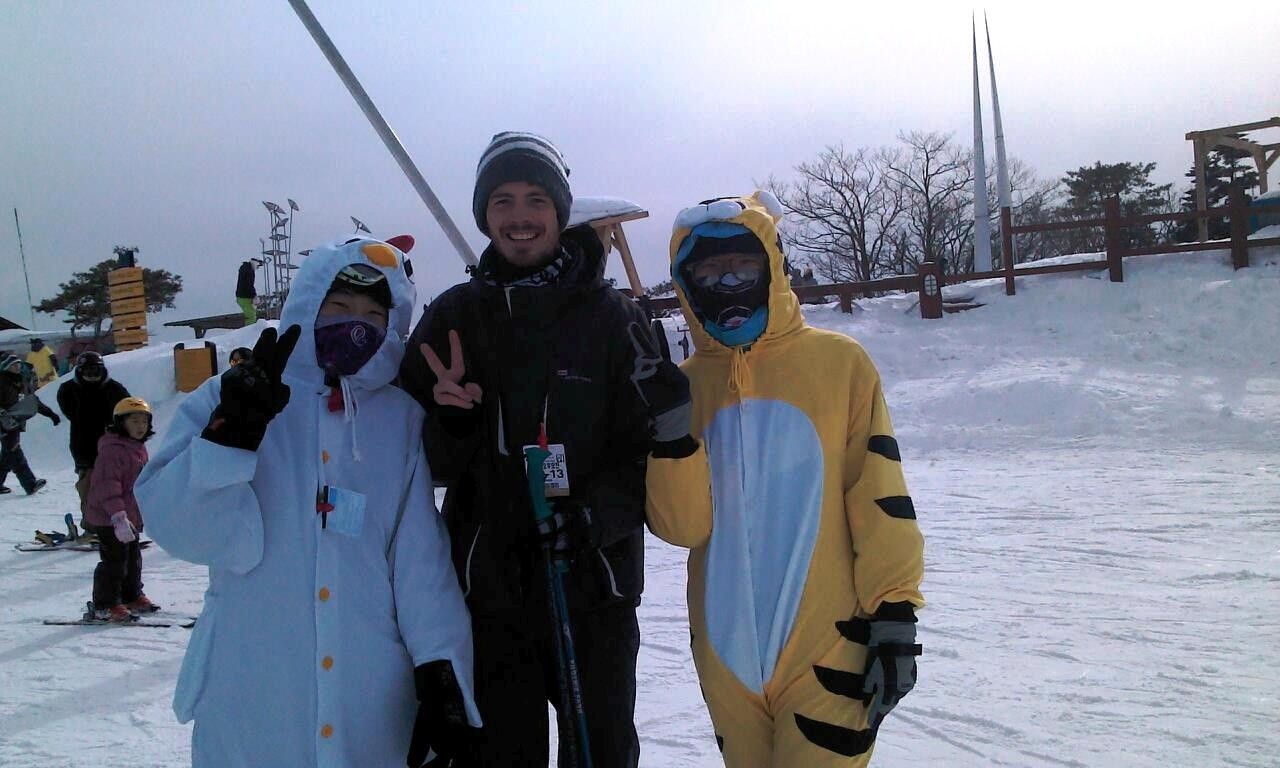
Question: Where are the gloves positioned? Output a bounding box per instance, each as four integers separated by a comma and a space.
112, 511, 138, 543
629, 316, 700, 458
0, 410, 19, 431
51, 414, 59, 427
862, 619, 922, 730
200, 323, 302, 452
407, 658, 474, 768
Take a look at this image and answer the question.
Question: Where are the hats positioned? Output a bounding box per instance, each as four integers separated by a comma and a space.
472, 131, 573, 239
331, 266, 391, 308
0, 351, 21, 370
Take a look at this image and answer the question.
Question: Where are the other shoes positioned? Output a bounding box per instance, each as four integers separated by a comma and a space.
125, 592, 159, 613
0, 485, 10, 494
96, 603, 140, 622
27, 478, 46, 496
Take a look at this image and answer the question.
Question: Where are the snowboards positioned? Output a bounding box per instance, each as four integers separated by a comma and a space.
0, 394, 38, 440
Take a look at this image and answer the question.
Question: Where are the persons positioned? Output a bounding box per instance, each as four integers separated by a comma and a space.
235, 255, 264, 325
26, 338, 59, 388
85, 398, 162, 622
56, 352, 132, 540
790, 268, 823, 303
134, 236, 482, 768
633, 192, 925, 768
0, 349, 61, 496
229, 347, 253, 367
392, 131, 652, 768
58, 350, 79, 377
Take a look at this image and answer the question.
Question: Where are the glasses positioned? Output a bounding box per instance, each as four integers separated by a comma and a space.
685, 254, 769, 279
229, 358, 243, 364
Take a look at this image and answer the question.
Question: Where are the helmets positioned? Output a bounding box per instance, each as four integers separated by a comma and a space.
76, 351, 104, 369
112, 398, 151, 422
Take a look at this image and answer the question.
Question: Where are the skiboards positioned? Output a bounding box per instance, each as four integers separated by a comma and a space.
43, 605, 201, 628
15, 534, 153, 553
